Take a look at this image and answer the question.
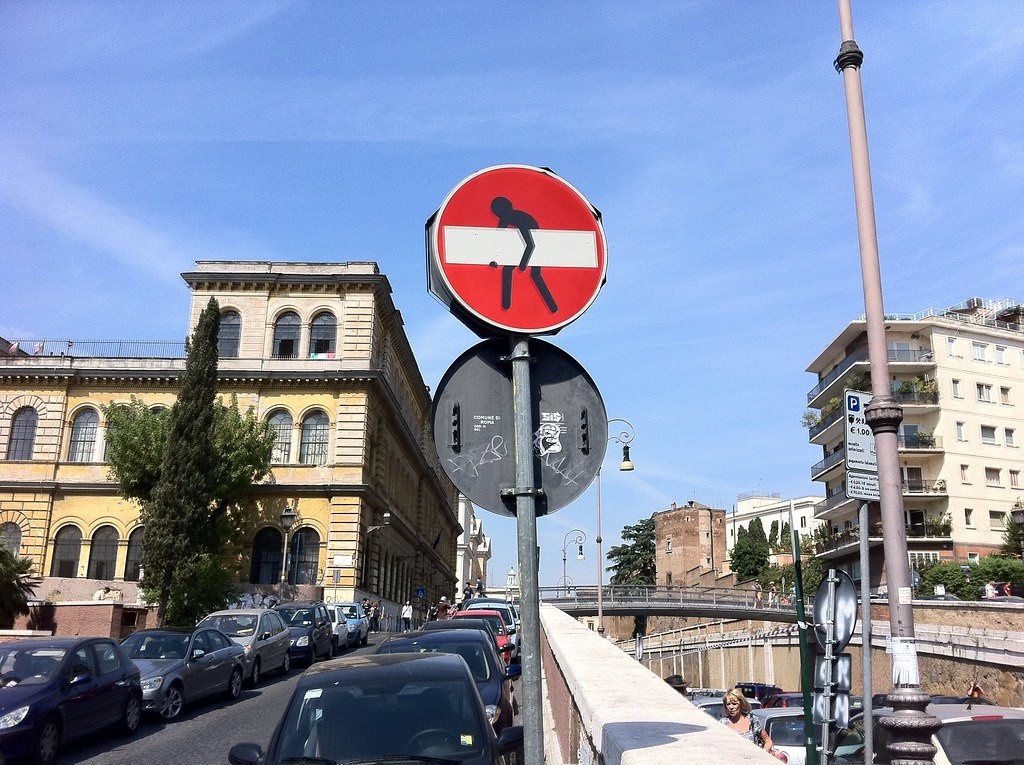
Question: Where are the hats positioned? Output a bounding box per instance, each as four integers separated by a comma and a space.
989, 581, 997, 584
663, 675, 692, 687
373, 602, 379, 606
441, 596, 447, 601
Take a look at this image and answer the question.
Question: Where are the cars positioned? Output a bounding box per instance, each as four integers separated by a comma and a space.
367, 629, 523, 739
826, 703, 1024, 765
292, 606, 349, 655
0, 636, 144, 765
856, 589, 962, 601
227, 652, 525, 765
183, 608, 291, 686
985, 595, 1024, 603
326, 603, 369, 648
105, 627, 247, 722
665, 672, 1001, 765
420, 599, 521, 667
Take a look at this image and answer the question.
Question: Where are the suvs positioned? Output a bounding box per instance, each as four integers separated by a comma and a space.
976, 580, 1024, 601
270, 600, 332, 667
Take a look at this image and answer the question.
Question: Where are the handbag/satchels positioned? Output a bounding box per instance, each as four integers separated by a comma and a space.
750, 713, 789, 765
401, 614, 403, 619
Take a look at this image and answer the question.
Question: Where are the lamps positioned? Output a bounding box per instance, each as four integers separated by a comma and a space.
396, 546, 423, 562
434, 578, 450, 589
364, 510, 391, 536
423, 563, 439, 577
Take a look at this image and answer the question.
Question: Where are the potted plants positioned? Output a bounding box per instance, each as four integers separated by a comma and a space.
914, 432, 936, 450
896, 375, 939, 405
922, 514, 952, 538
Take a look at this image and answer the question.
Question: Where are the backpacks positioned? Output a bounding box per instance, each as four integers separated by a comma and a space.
373, 606, 380, 618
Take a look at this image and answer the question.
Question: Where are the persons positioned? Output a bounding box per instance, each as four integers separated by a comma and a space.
717, 689, 773, 753
768, 581, 779, 609
789, 581, 797, 609
751, 578, 765, 609
967, 681, 985, 698
401, 600, 413, 633
357, 598, 384, 633
434, 596, 451, 621
984, 578, 996, 600
92, 587, 110, 600
1004, 581, 1013, 596
426, 601, 438, 621
461, 581, 474, 605
470, 577, 485, 598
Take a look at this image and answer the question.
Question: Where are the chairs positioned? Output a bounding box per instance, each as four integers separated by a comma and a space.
390, 690, 460, 755
305, 692, 378, 763
142, 641, 158, 655
183, 641, 201, 657
224, 620, 237, 634
456, 647, 483, 677
3, 655, 30, 679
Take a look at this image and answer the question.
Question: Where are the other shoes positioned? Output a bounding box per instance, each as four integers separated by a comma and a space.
404, 630, 406, 633
408, 630, 411, 633
370, 630, 375, 633
376, 631, 380, 633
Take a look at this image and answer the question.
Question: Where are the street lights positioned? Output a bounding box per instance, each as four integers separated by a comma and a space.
561, 529, 587, 598
594, 417, 636, 637
556, 576, 574, 599
